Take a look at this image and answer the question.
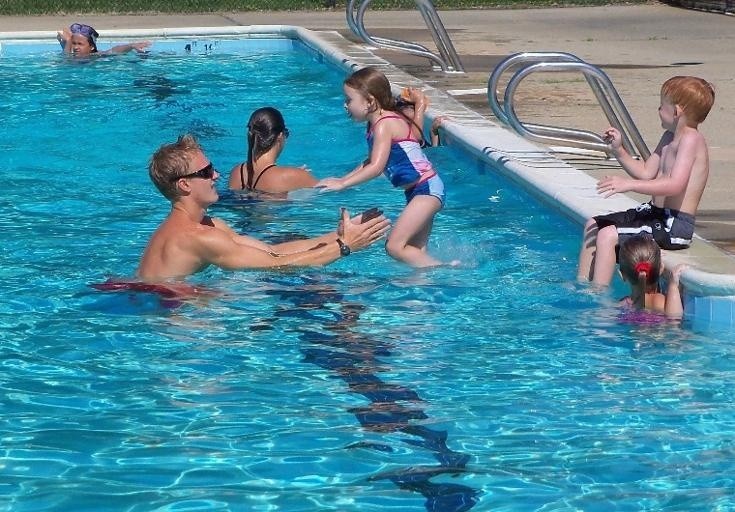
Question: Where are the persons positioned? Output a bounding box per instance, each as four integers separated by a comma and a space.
578, 75, 716, 285
617, 236, 685, 324
227, 107, 320, 198
314, 67, 461, 272
56, 22, 152, 64
135, 132, 393, 287
395, 85, 449, 147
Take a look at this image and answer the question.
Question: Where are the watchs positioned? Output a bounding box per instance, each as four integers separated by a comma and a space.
336, 238, 350, 256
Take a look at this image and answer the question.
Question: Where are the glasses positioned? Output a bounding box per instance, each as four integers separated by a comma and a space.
70, 23, 97, 37
175, 162, 213, 179
